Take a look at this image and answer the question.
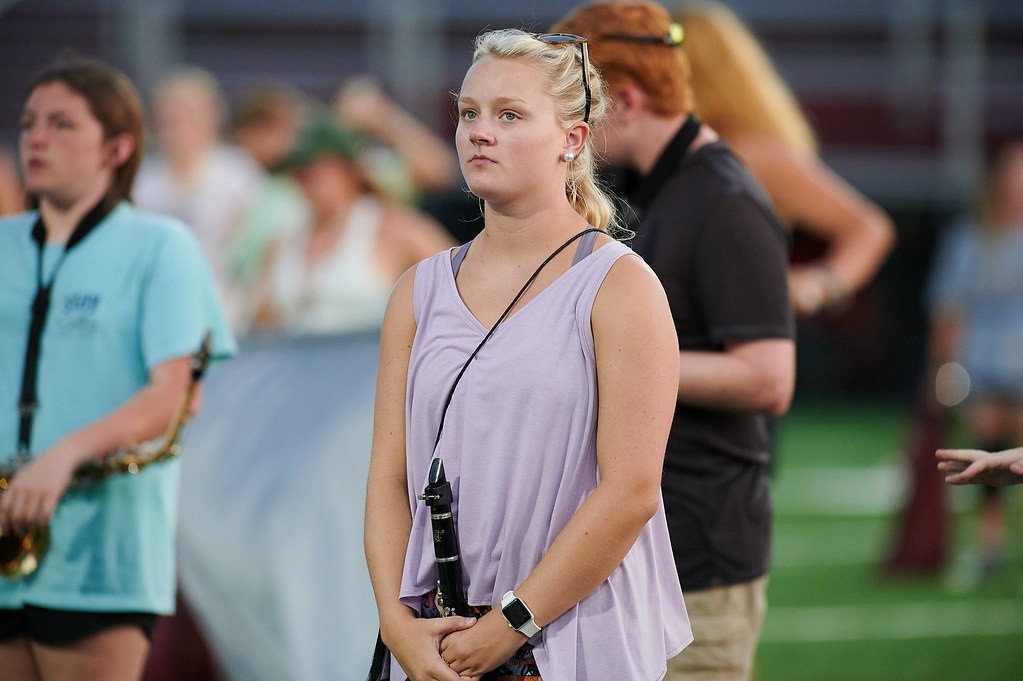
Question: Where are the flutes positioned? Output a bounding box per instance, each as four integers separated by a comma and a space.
415, 455, 471, 619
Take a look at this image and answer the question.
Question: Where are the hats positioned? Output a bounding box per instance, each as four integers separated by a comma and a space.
268, 112, 368, 172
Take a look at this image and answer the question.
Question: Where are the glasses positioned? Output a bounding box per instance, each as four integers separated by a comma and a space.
601, 23, 685, 47
529, 32, 592, 123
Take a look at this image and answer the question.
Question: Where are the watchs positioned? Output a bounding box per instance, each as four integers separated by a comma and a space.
500, 591, 542, 638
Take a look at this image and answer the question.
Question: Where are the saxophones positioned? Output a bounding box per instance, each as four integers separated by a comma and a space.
0, 326, 223, 582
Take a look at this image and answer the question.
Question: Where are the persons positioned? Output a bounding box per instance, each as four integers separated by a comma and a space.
918, 134, 1023, 569
363, 25, 694, 681
1, 60, 240, 679
0, 0, 895, 681
